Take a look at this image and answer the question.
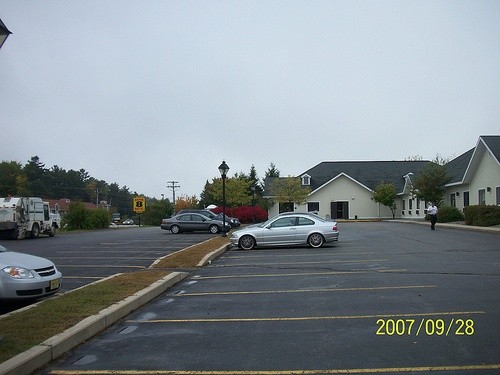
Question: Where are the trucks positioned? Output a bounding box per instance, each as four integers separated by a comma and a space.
0, 195, 61, 240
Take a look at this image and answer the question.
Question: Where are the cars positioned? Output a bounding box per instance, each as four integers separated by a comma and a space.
123, 219, 135, 225
0, 244, 63, 301
161, 212, 232, 234
228, 211, 340, 249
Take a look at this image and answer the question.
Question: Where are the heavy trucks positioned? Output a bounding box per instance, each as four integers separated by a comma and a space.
112, 213, 121, 223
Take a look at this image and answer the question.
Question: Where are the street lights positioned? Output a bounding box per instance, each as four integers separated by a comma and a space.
217, 160, 231, 237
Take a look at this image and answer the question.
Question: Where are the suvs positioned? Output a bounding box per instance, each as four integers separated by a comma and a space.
175, 209, 241, 228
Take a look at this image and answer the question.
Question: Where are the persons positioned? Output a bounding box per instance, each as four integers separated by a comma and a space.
427, 202, 438, 230
